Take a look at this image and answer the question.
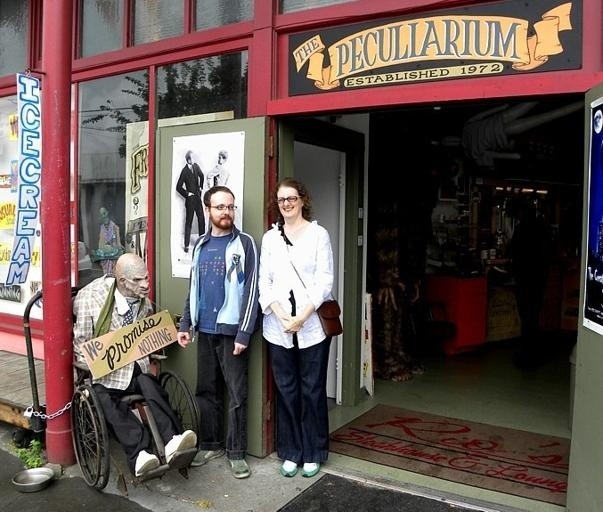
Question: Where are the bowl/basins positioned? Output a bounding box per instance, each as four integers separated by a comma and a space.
8, 467, 56, 493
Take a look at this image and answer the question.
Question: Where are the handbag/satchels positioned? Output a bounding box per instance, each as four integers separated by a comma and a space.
317, 299, 344, 336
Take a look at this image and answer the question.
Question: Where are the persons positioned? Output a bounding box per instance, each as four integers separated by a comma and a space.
176, 151, 205, 252
257, 177, 335, 477
497, 191, 562, 371
98, 207, 122, 276
206, 152, 230, 233
72, 252, 197, 479
177, 186, 260, 478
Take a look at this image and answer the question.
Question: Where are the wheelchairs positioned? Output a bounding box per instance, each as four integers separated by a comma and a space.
67, 288, 204, 501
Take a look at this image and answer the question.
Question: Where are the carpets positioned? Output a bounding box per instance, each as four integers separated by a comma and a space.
277, 473, 486, 512
327, 402, 571, 507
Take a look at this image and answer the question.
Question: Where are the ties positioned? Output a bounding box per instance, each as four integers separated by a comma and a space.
122, 302, 132, 327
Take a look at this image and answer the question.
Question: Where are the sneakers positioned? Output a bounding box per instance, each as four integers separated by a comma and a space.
134, 450, 161, 479
280, 460, 298, 478
227, 457, 251, 479
301, 462, 321, 477
191, 449, 226, 466
164, 430, 197, 463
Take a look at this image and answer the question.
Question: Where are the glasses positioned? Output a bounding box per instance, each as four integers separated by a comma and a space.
276, 195, 302, 203
213, 205, 237, 210
